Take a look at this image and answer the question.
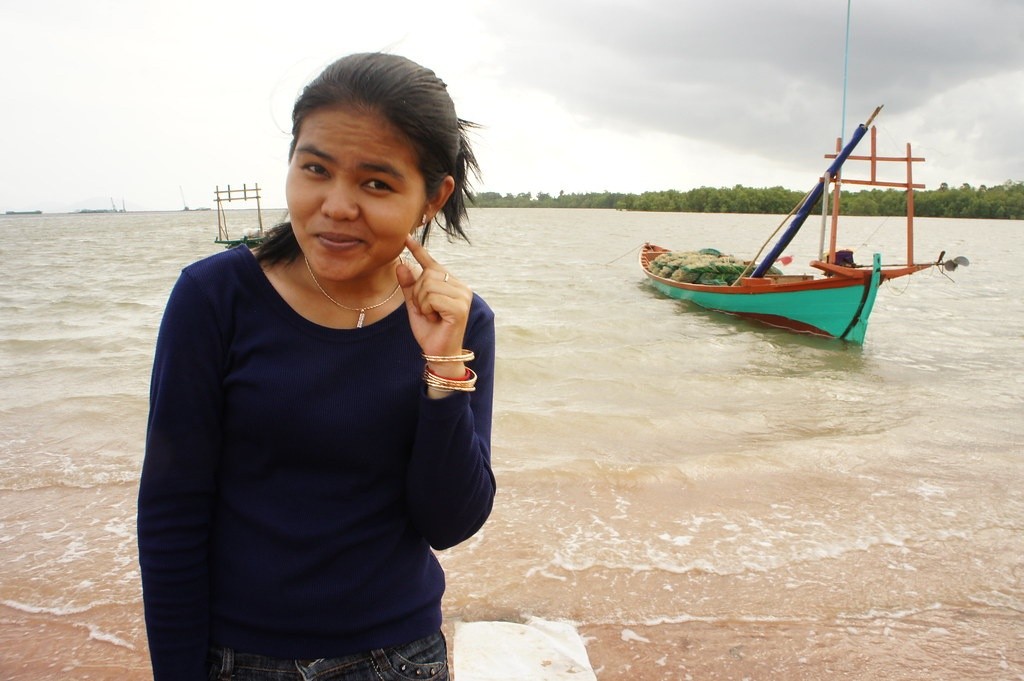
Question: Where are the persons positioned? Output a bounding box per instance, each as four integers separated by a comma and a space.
136, 52, 497, 681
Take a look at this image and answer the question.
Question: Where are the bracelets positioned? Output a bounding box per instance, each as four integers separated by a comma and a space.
419, 349, 479, 393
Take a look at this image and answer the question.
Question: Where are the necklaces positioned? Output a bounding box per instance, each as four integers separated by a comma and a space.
304, 255, 405, 327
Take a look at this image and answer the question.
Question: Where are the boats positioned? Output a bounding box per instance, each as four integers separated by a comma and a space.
638, 101, 932, 349
212, 182, 271, 251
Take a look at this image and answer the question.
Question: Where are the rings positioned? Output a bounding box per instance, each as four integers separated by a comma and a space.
444, 273, 449, 282
